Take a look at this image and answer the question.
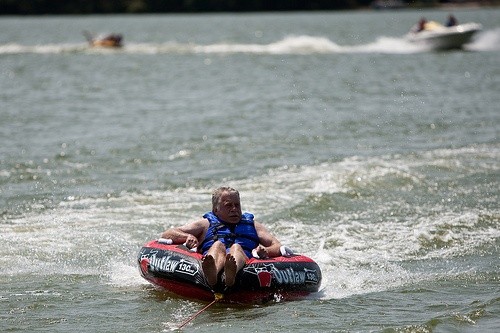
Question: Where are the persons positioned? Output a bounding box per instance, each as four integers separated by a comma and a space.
444, 13, 458, 27
411, 19, 426, 33
162, 186, 285, 286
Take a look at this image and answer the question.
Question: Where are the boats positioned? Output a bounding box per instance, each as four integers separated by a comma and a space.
406, 22, 483, 51
